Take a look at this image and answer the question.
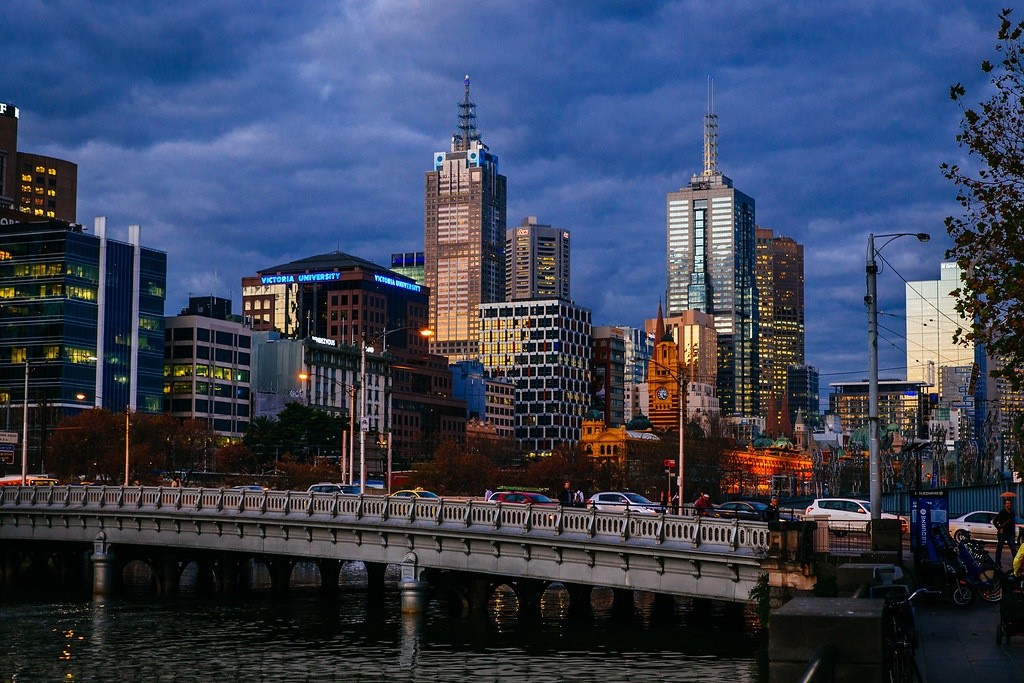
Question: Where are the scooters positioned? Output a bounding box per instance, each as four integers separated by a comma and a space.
694, 506, 717, 518
940, 535, 1003, 608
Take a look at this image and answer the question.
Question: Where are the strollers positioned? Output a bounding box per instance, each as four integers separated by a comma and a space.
996, 567, 1024, 645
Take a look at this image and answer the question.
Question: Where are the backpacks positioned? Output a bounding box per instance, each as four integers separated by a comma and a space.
574, 491, 582, 504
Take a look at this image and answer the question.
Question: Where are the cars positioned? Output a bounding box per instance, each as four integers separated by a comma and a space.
306, 484, 362, 495
232, 485, 271, 492
487, 491, 561, 507
586, 491, 663, 515
383, 489, 439, 498
949, 510, 1024, 545
714, 501, 798, 522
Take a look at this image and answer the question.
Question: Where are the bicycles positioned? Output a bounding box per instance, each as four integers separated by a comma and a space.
883, 587, 925, 683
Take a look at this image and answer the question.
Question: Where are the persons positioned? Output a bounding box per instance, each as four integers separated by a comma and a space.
1012, 542, 1024, 577
575, 487, 584, 503
766, 498, 779, 521
994, 499, 1018, 568
172, 476, 183, 487
485, 488, 492, 501
662, 492, 667, 505
695, 493, 712, 517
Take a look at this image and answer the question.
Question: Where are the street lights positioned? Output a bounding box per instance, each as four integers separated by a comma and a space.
360, 325, 435, 490
864, 232, 931, 538
631, 356, 685, 515
75, 393, 130, 485
298, 372, 355, 486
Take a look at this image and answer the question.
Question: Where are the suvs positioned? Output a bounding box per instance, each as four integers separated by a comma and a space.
806, 497, 908, 538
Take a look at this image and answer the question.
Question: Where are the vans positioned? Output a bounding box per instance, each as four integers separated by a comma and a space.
352, 480, 386, 489
28, 477, 66, 486
78, 472, 118, 487
